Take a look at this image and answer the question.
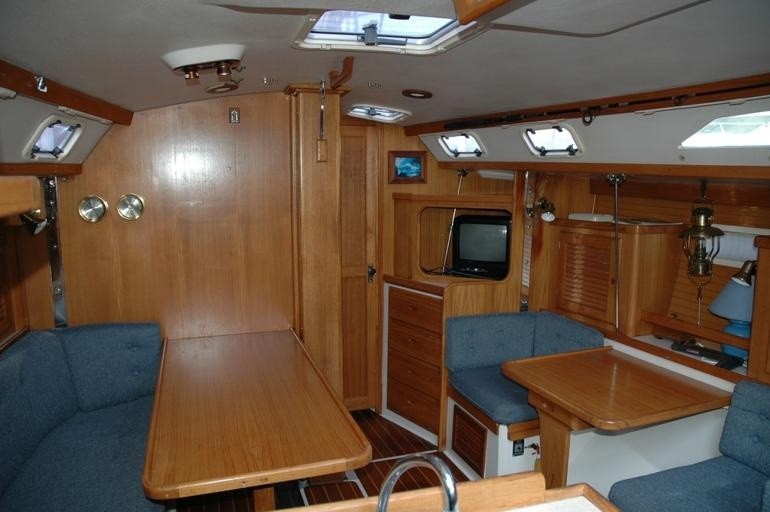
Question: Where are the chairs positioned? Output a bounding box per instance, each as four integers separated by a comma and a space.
443, 311, 605, 442
609, 377, 770, 511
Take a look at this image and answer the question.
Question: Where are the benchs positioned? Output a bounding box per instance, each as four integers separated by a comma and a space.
1, 320, 163, 512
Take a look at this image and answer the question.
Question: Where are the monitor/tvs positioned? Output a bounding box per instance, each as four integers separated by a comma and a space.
451, 214, 511, 281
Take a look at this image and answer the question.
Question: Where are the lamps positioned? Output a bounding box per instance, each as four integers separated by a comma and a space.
526, 195, 556, 222
20, 210, 48, 235
677, 178, 757, 365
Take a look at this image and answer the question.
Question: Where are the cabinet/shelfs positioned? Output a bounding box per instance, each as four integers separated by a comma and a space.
549, 219, 690, 337
383, 286, 521, 436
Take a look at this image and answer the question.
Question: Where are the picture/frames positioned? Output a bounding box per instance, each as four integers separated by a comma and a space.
387, 149, 429, 185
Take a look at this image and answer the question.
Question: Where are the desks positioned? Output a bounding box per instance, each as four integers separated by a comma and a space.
500, 347, 733, 490
140, 327, 373, 512
